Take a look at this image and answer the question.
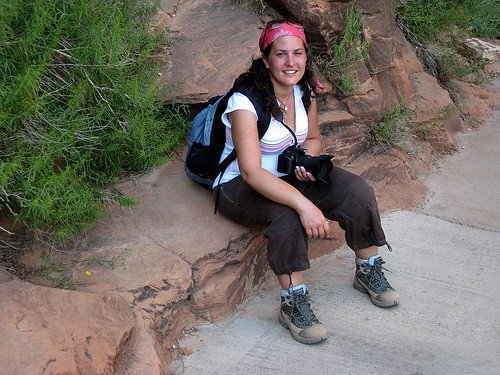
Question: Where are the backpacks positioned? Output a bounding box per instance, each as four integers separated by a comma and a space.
185, 84, 309, 192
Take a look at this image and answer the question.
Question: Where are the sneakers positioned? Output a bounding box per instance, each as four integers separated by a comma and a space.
279, 284, 327, 344
353, 255, 400, 308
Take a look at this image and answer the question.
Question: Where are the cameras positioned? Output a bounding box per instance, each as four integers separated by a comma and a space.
278, 146, 335, 184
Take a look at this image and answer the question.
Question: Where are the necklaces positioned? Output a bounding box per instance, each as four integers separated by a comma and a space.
275, 92, 292, 114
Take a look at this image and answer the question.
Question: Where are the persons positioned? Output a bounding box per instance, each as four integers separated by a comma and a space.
212, 20, 401, 344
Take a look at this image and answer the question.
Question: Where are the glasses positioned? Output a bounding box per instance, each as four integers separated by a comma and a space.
262, 19, 303, 48
